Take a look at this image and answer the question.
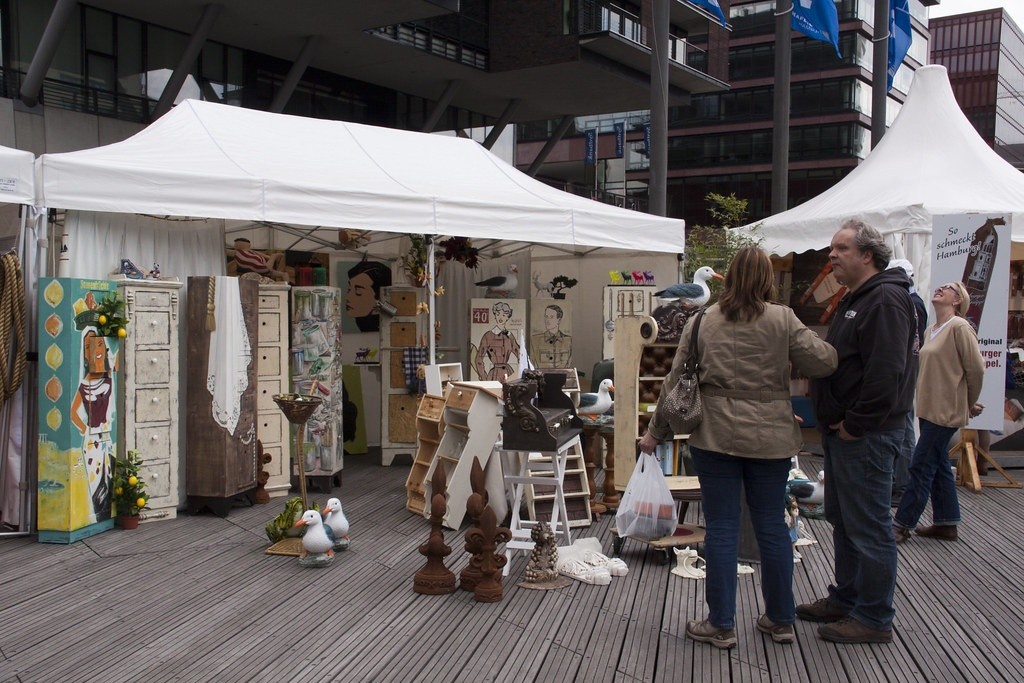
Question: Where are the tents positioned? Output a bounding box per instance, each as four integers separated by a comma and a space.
725, 64, 1024, 476
0, 99, 685, 539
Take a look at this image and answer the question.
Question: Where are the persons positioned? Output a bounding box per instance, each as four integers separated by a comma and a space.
890, 280, 984, 542
795, 220, 916, 642
639, 247, 838, 650
882, 260, 928, 507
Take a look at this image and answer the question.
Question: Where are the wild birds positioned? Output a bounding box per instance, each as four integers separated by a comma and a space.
652, 265, 725, 306
295, 510, 333, 558
321, 498, 350, 541
790, 470, 824, 510
576, 378, 615, 421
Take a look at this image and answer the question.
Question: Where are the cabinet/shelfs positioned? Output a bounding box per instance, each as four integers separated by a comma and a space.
113, 269, 703, 530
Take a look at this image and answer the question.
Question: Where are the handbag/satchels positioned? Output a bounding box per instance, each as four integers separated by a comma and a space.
616, 452, 678, 541
662, 307, 706, 433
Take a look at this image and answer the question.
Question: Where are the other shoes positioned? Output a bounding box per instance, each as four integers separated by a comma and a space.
893, 525, 911, 543
916, 523, 958, 540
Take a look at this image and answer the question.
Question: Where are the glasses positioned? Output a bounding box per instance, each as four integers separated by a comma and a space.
935, 285, 961, 297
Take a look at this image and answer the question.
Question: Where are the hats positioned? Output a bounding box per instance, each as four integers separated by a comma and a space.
885, 258, 914, 278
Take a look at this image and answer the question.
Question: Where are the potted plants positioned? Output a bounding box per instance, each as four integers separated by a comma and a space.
111, 449, 150, 528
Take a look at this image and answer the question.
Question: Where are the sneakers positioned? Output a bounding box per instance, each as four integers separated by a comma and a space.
795, 598, 845, 622
687, 619, 736, 649
817, 616, 893, 643
757, 613, 795, 643
